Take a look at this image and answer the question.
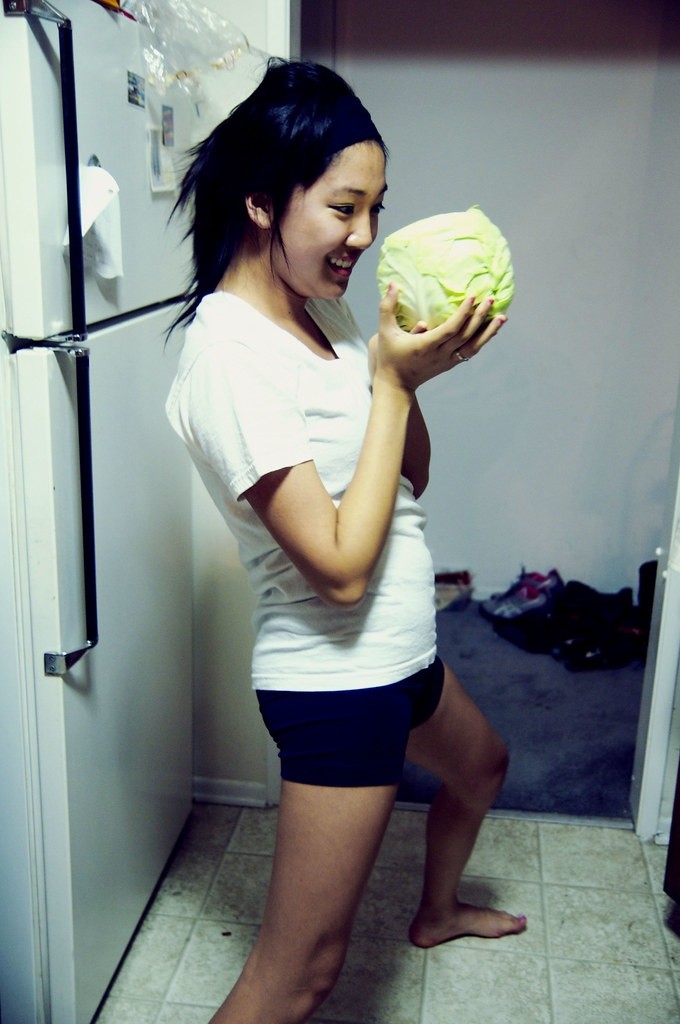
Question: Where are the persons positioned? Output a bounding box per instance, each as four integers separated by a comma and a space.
163, 58, 528, 1024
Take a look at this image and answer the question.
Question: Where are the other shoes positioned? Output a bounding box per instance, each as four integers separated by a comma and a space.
478, 563, 565, 622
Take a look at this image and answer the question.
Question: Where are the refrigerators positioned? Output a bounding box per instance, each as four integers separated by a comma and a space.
0, 0, 200, 1024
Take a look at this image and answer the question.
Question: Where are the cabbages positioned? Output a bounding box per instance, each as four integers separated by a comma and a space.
376, 203, 514, 332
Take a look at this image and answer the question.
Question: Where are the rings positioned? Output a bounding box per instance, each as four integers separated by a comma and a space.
455, 351, 469, 361
